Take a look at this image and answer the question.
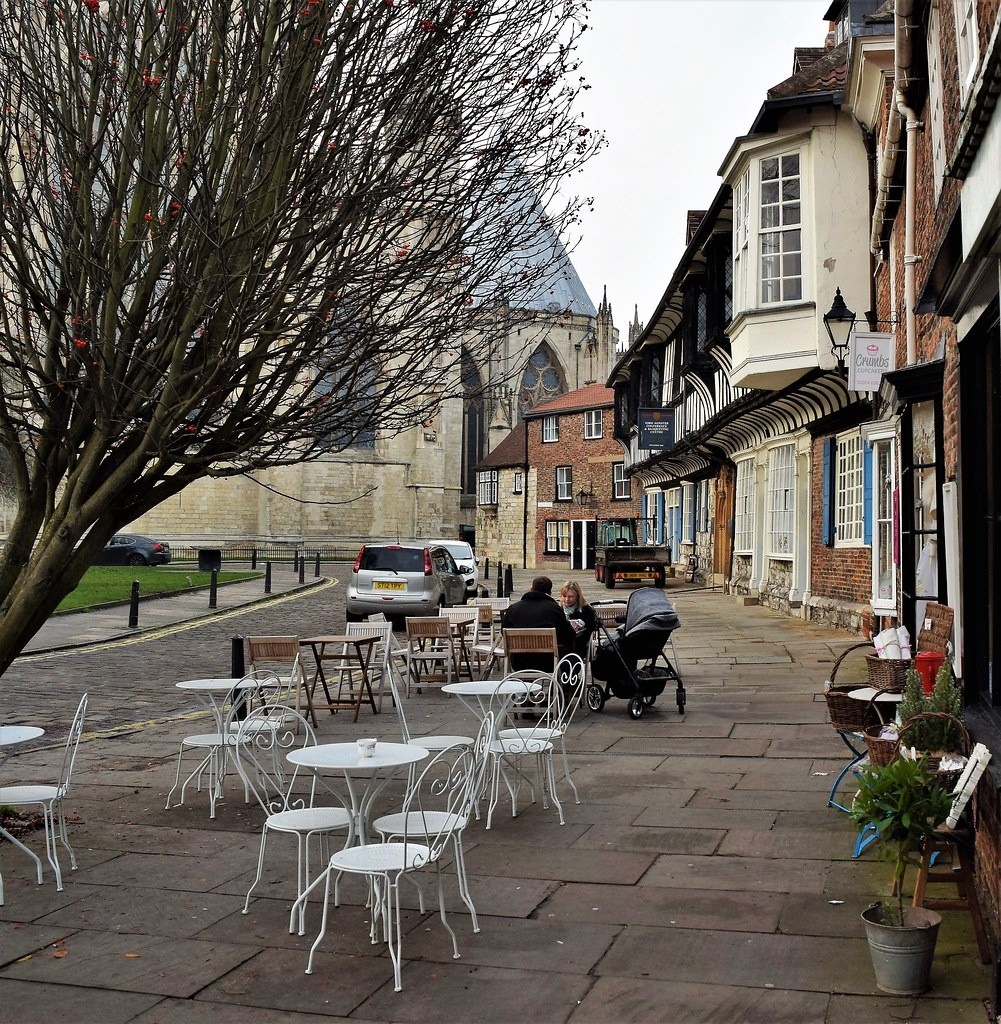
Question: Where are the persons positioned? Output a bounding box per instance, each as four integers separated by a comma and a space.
558, 579, 596, 706
501, 575, 578, 720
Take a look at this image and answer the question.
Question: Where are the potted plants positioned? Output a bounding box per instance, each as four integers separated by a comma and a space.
845, 751, 960, 997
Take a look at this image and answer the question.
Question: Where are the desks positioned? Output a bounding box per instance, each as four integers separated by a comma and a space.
285, 742, 429, 946
415, 619, 474, 681
298, 635, 381, 723
174, 679, 272, 806
441, 680, 542, 818
0, 727, 44, 906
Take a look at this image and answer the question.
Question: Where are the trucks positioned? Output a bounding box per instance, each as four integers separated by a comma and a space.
595, 517, 672, 589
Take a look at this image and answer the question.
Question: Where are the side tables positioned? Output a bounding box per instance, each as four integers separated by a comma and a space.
915, 601, 954, 653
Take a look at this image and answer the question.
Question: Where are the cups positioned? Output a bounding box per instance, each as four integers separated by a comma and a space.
357, 739, 376, 758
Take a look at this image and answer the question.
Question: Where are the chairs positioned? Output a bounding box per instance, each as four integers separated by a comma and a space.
163, 596, 681, 993
0, 692, 87, 893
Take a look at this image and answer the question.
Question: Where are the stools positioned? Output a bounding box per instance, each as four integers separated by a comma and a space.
891, 826, 992, 967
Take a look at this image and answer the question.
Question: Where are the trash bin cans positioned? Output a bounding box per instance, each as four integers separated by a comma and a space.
198, 550, 221, 572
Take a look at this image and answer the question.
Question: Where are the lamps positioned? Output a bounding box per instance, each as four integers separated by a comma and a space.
575, 490, 594, 506
822, 286, 858, 379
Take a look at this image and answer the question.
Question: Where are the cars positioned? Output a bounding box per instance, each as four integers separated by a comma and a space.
90, 533, 171, 566
426, 539, 480, 598
345, 540, 469, 623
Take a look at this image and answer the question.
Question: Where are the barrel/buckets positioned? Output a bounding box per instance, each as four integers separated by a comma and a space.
916, 653, 945, 696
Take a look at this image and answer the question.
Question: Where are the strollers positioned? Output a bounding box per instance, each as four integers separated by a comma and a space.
585, 587, 686, 720
